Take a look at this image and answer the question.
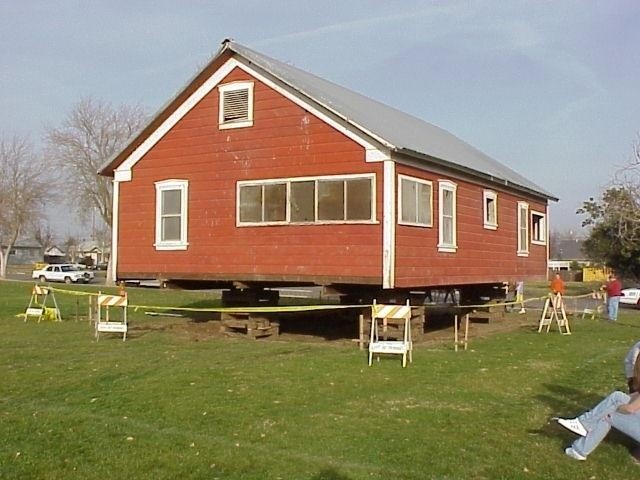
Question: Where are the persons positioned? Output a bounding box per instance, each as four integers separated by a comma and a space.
599, 275, 622, 321
557, 340, 640, 461
550, 272, 565, 296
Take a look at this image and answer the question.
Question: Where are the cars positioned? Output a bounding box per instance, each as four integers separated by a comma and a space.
31, 264, 94, 285
619, 288, 640, 309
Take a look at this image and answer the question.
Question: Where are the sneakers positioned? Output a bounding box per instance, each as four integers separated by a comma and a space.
565, 447, 586, 461
558, 417, 587, 437
